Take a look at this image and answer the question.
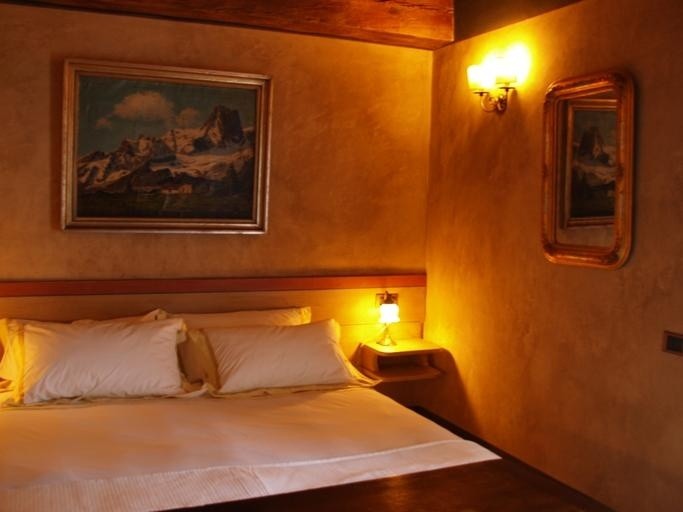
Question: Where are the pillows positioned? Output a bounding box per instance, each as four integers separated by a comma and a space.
172, 306, 312, 382
8, 319, 187, 399
1, 309, 167, 352
188, 317, 376, 397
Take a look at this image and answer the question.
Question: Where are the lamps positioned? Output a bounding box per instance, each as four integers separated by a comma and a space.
467, 41, 531, 113
374, 289, 401, 346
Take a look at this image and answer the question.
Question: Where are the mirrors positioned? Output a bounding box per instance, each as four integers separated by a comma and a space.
539, 68, 636, 269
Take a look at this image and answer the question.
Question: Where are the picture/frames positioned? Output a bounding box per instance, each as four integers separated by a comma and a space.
61, 58, 275, 236
559, 99, 618, 231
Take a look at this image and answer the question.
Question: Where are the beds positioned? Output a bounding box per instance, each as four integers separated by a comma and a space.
0, 274, 505, 512
